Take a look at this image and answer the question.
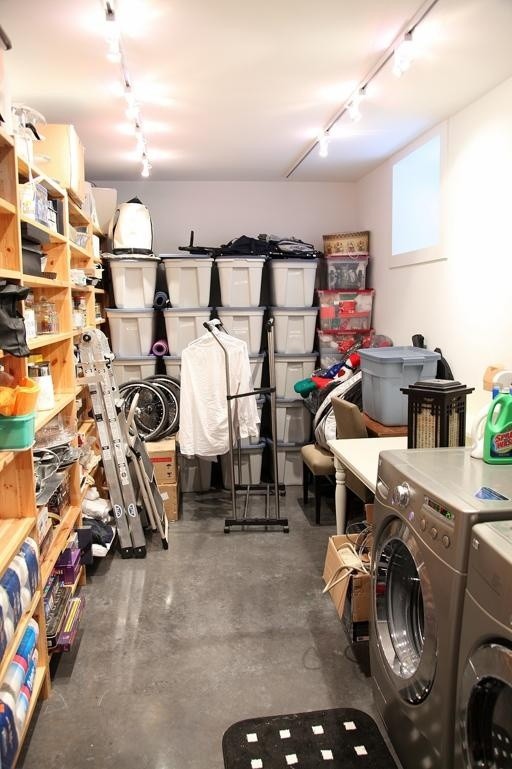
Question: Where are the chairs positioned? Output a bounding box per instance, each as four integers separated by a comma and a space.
329, 396, 376, 504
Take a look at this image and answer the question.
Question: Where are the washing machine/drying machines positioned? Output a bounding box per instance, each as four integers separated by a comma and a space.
451, 519, 512, 769
367, 446, 512, 768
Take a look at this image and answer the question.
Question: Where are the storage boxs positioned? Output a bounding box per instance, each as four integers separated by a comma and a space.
358, 345, 441, 428
100, 255, 319, 522
320, 532, 379, 661
0, 122, 88, 206
317, 252, 375, 369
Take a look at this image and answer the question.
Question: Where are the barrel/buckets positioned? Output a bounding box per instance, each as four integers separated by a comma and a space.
469, 386, 498, 461
484, 384, 512, 465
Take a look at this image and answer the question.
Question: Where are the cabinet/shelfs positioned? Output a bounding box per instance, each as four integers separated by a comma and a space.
0, 135, 117, 769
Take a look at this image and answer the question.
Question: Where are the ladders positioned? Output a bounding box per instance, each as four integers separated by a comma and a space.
80, 328, 169, 559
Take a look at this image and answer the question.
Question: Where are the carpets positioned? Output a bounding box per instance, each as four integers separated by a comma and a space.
222, 707, 398, 768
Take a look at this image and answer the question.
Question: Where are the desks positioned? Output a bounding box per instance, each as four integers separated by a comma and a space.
359, 410, 409, 436
358, 410, 409, 437
324, 436, 409, 535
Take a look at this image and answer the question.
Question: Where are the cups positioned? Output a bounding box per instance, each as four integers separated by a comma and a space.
27, 361, 55, 410
31, 302, 57, 335
